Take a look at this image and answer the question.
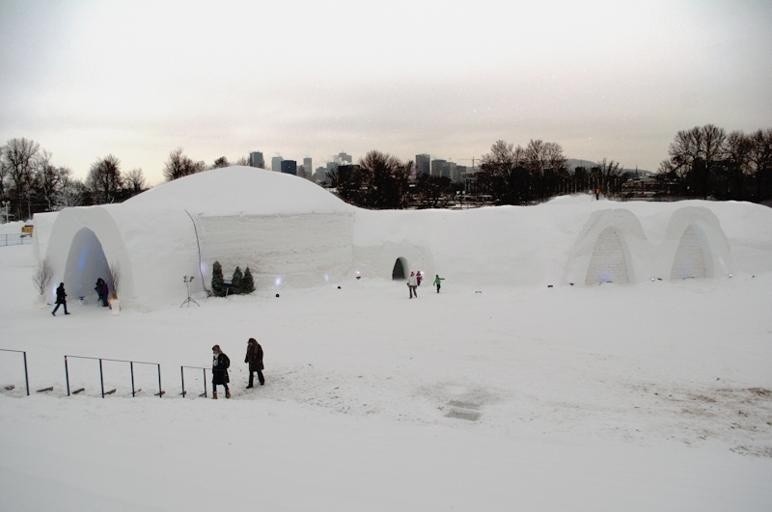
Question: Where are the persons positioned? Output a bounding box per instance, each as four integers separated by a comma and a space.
416, 271, 422, 286
52, 282, 70, 316
212, 345, 230, 398
244, 339, 264, 389
595, 187, 600, 200
407, 271, 417, 299
433, 275, 445, 293
95, 278, 109, 307
